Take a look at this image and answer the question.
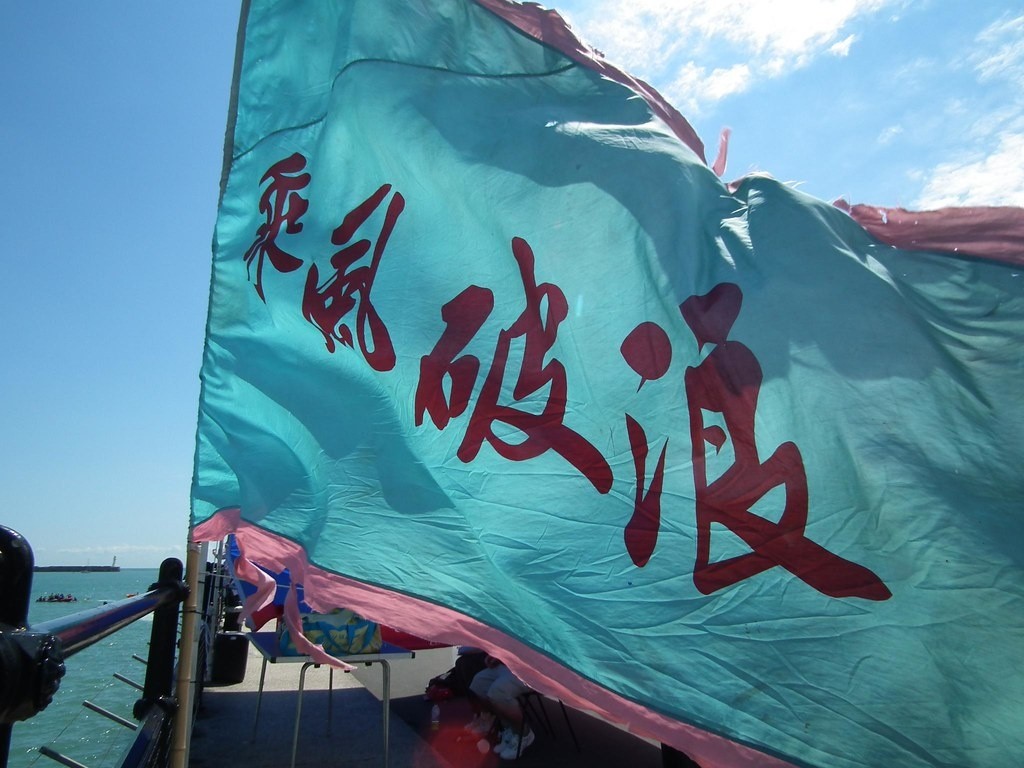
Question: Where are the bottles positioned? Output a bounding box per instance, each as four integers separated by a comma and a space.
430, 703, 440, 730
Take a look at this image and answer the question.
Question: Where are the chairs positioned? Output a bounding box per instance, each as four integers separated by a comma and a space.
485, 687, 587, 758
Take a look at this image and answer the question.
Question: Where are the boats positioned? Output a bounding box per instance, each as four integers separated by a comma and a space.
36, 597, 72, 600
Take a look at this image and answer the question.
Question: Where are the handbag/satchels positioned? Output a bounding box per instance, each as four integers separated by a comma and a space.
430, 676, 446, 688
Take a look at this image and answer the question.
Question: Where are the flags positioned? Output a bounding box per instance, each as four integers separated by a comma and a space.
186, 0, 1023, 768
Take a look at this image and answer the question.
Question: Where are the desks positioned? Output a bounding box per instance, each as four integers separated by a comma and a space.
242, 630, 417, 767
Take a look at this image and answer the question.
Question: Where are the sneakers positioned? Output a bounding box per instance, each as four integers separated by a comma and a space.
492, 727, 535, 760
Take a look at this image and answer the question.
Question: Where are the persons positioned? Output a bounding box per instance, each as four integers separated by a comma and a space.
40, 593, 72, 599
456, 650, 495, 735
471, 654, 536, 760
